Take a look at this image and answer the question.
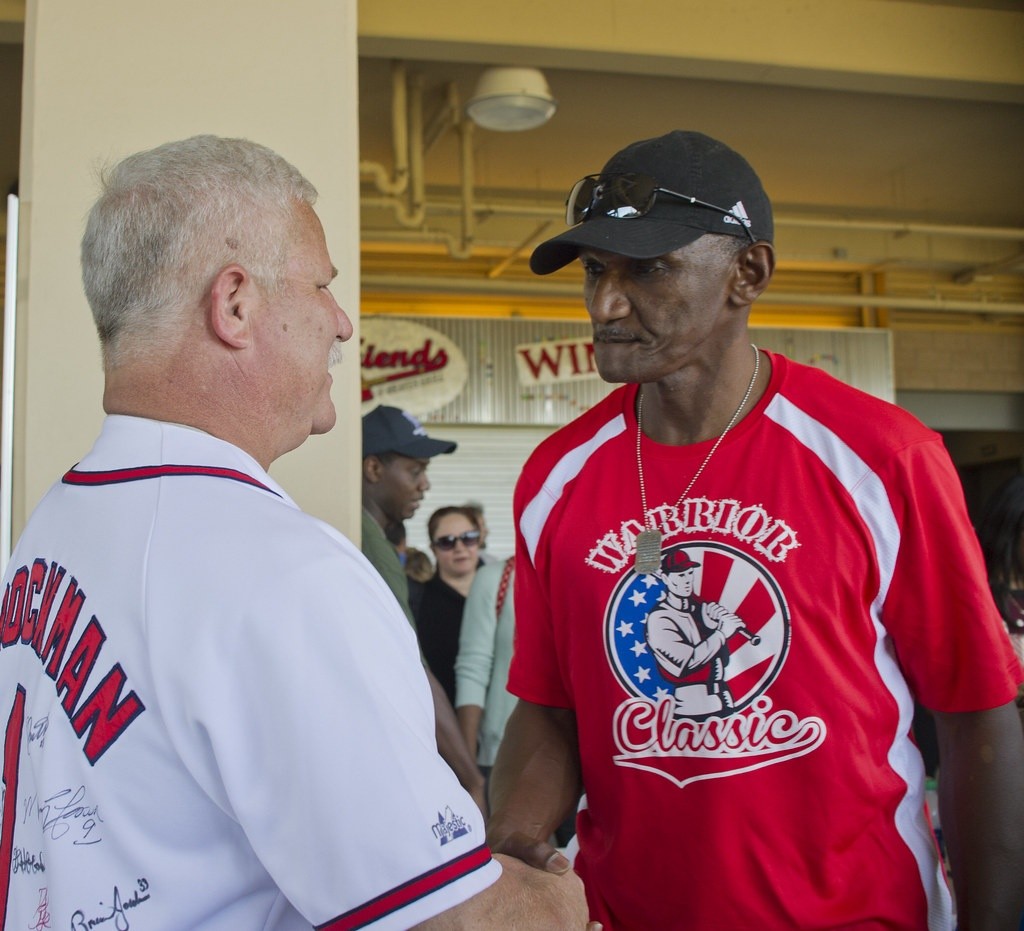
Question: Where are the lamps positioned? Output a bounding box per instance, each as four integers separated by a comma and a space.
466, 65, 558, 132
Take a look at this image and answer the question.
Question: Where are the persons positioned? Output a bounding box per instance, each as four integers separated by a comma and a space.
911, 481, 1024, 879
362, 404, 577, 850
490, 131, 1024, 930
0, 139, 601, 931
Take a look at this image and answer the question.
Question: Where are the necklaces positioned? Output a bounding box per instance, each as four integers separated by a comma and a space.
636, 342, 760, 575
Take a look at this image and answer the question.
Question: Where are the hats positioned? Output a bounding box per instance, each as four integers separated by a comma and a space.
362, 405, 457, 461
530, 130, 774, 276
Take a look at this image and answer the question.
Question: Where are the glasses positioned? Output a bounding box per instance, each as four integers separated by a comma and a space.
432, 529, 480, 551
565, 173, 756, 246
